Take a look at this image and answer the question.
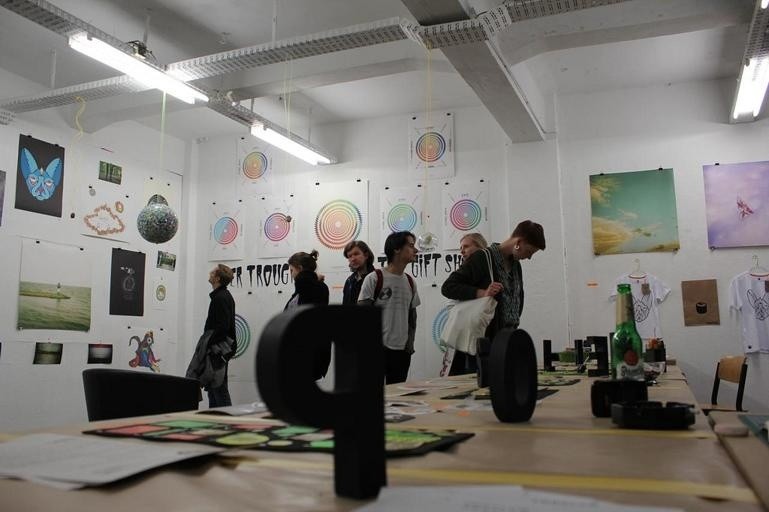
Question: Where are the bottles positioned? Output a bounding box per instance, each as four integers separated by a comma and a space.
610, 282, 646, 381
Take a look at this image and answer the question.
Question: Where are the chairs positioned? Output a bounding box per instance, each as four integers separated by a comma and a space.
693, 352, 752, 415
76, 362, 205, 424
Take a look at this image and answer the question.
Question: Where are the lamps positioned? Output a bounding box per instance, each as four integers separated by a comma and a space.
727, 1, 768, 122
64, 8, 341, 170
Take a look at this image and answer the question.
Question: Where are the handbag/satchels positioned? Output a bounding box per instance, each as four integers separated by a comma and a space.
440, 295, 498, 359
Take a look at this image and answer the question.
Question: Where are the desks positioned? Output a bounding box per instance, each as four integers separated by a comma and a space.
705, 404, 769, 510
0, 351, 765, 511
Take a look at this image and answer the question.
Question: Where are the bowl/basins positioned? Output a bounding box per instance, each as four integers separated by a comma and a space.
641, 360, 667, 385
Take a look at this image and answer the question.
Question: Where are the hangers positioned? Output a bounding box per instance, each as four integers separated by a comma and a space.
628, 258, 648, 279
747, 254, 769, 278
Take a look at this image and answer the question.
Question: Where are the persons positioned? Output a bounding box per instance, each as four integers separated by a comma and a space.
441, 221, 546, 386
442, 234, 487, 377
281, 250, 330, 380
206, 263, 236, 408
343, 240, 376, 305
358, 231, 422, 384
